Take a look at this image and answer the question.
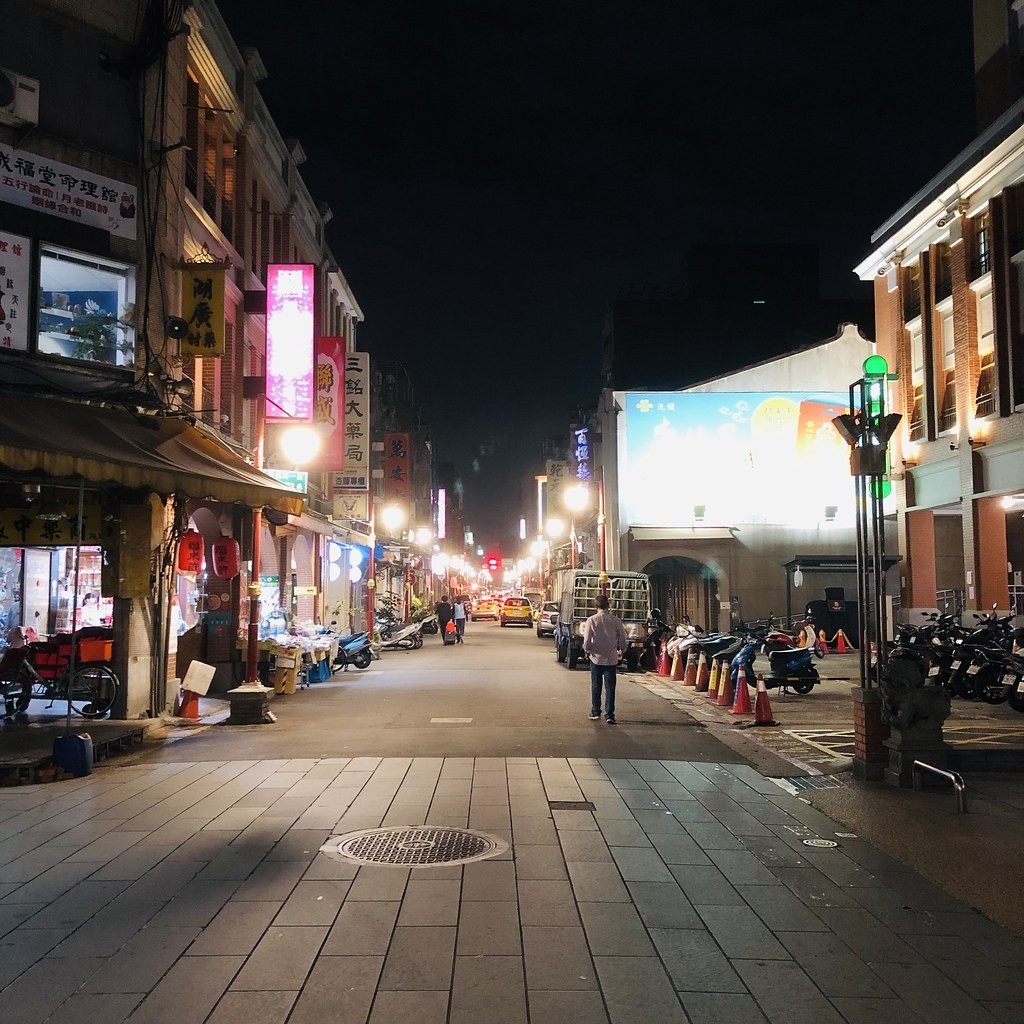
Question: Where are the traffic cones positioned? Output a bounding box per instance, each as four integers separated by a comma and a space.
680, 648, 697, 687
692, 651, 710, 692
748, 673, 780, 727
727, 666, 755, 716
655, 638, 666, 672
704, 658, 720, 699
668, 644, 685, 682
819, 630, 830, 654
713, 659, 735, 706
174, 689, 203, 720
835, 629, 852, 655
655, 643, 670, 678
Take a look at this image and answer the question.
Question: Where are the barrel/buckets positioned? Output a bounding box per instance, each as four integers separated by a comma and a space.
54, 732, 94, 775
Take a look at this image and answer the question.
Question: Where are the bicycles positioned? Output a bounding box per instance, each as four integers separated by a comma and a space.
294, 630, 347, 674
0, 636, 119, 722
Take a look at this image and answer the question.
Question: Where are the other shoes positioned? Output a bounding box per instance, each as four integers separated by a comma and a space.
460, 637, 464, 644
587, 711, 600, 720
606, 716, 618, 725
456, 637, 460, 644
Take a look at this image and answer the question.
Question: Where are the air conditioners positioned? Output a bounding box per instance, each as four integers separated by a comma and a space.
0, 66, 40, 128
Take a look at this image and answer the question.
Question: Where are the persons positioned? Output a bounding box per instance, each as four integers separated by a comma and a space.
451, 597, 468, 643
582, 595, 626, 723
83, 593, 100, 624
435, 595, 455, 645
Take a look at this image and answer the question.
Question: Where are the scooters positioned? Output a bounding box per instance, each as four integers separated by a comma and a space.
373, 608, 439, 652
871, 599, 1024, 717
728, 624, 822, 698
643, 605, 825, 677
314, 616, 379, 669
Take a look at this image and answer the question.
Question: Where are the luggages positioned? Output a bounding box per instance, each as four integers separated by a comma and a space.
445, 631, 455, 645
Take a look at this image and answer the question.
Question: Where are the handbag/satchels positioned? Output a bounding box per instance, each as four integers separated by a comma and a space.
446, 621, 456, 632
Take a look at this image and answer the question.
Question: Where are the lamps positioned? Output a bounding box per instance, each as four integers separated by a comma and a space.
968, 437, 986, 446
950, 442, 958, 450
35, 488, 68, 521
825, 506, 838, 521
694, 506, 705, 520
165, 377, 194, 400
165, 317, 190, 339
902, 458, 916, 466
165, 135, 193, 152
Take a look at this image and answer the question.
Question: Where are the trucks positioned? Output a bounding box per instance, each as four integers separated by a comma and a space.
550, 567, 654, 670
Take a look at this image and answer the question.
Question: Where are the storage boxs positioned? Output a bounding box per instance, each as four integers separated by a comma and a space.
31, 633, 113, 677
235, 641, 325, 662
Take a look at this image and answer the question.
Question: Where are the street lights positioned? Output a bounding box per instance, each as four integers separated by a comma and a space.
239, 390, 324, 686
544, 519, 579, 569
561, 463, 609, 595
404, 514, 433, 625
362, 473, 407, 660
831, 377, 905, 692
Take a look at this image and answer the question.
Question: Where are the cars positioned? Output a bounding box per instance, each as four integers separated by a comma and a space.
451, 587, 546, 629
535, 601, 560, 638
